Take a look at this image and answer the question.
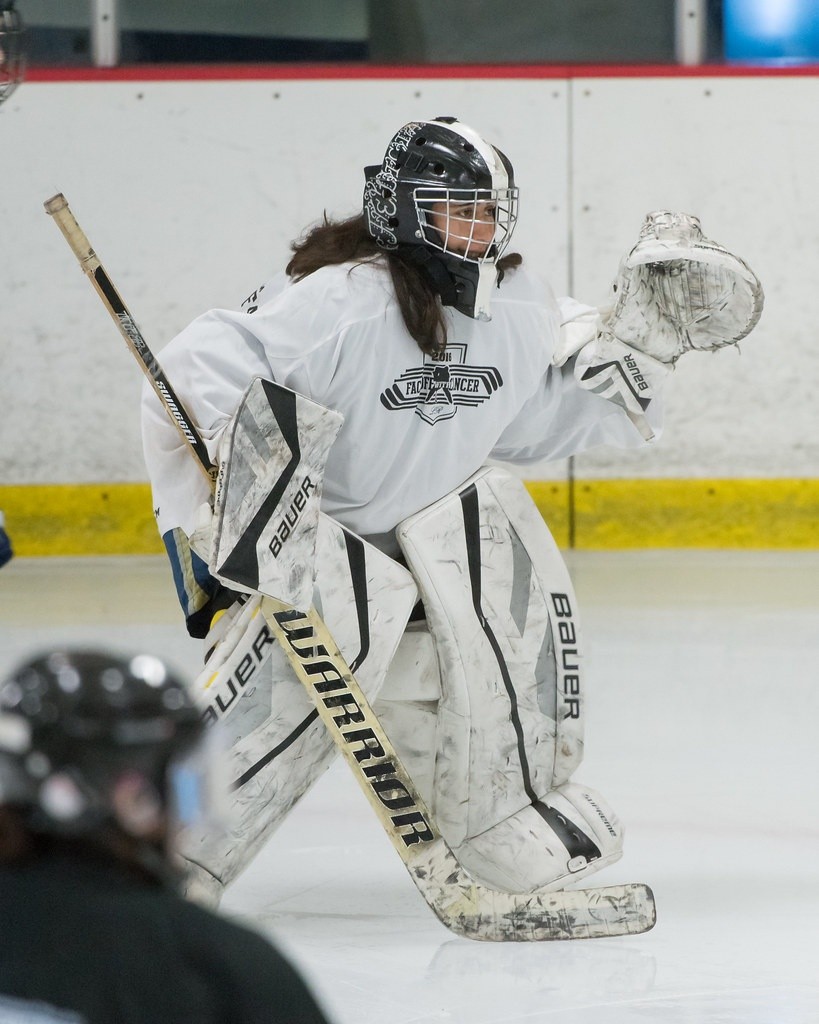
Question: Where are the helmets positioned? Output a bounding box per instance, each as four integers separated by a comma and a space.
364, 115, 520, 321
0, 646, 208, 783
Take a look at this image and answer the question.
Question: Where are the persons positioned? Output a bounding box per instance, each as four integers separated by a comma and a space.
140, 117, 764, 911
0, 647, 332, 1024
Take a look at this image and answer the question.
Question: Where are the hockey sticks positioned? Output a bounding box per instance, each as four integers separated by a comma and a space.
44, 192, 658, 942
